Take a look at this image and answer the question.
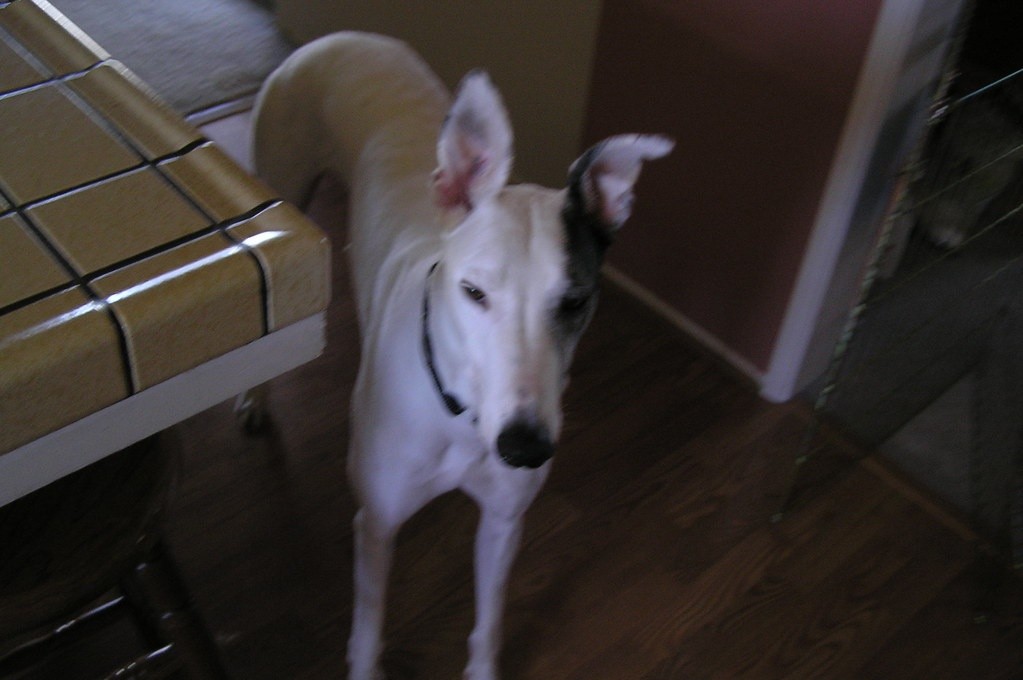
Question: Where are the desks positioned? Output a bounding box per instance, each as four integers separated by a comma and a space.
0, 0, 332, 680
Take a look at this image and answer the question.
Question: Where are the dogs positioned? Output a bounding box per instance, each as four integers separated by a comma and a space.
249, 30, 676, 680
